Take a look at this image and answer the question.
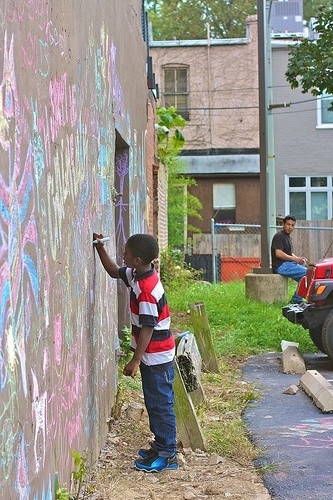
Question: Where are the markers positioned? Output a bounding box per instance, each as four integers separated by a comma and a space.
92, 237, 113, 244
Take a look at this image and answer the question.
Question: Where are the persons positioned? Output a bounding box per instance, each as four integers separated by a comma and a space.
271, 215, 309, 304
93, 232, 179, 473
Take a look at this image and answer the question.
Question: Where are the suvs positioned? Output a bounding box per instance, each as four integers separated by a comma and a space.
281, 257, 333, 360
